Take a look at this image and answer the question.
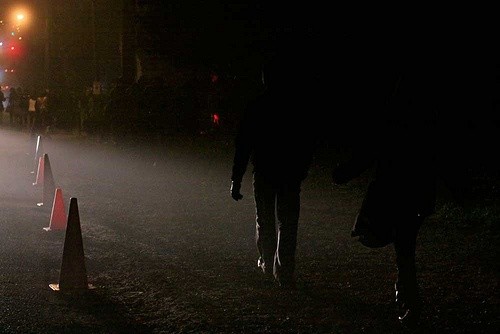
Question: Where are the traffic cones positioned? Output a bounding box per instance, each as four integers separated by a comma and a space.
30, 136, 45, 186
42, 188, 68, 233
49, 197, 96, 293
34, 153, 56, 211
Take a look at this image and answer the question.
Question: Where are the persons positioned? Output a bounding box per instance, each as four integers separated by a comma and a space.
231, 88, 313, 273
378, 67, 437, 304
0, 86, 55, 129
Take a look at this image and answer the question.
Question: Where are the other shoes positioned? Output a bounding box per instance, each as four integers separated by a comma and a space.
257, 256, 271, 276
398, 303, 421, 328
274, 271, 297, 283
395, 278, 419, 302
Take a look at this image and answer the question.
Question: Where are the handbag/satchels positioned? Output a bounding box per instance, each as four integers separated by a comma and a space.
352, 178, 393, 248
